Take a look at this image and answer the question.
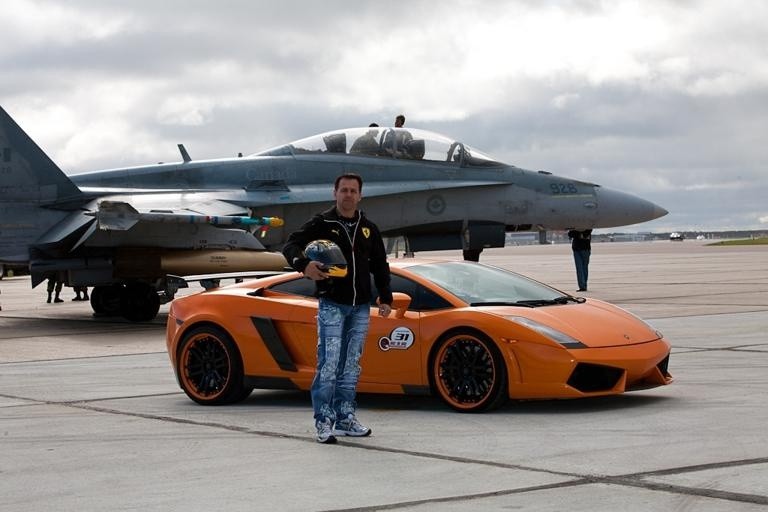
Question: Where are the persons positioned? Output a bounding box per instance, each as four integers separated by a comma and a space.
350, 124, 381, 155
569, 228, 593, 292
463, 248, 482, 261
282, 173, 393, 444
385, 114, 412, 143
72, 287, 90, 301
46, 280, 63, 304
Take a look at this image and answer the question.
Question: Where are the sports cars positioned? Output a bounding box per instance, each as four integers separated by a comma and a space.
165, 255, 675, 414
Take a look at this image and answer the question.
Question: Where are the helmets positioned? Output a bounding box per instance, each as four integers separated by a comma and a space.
304, 239, 348, 277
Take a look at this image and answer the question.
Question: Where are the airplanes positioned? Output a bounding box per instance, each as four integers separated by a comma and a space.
0, 106, 669, 324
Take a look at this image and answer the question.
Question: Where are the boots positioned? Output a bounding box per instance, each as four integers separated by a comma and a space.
47, 292, 52, 303
81, 290, 90, 301
71, 292, 81, 301
54, 291, 64, 302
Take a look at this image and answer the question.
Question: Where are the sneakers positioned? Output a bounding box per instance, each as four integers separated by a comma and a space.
316, 420, 338, 443
334, 416, 372, 437
